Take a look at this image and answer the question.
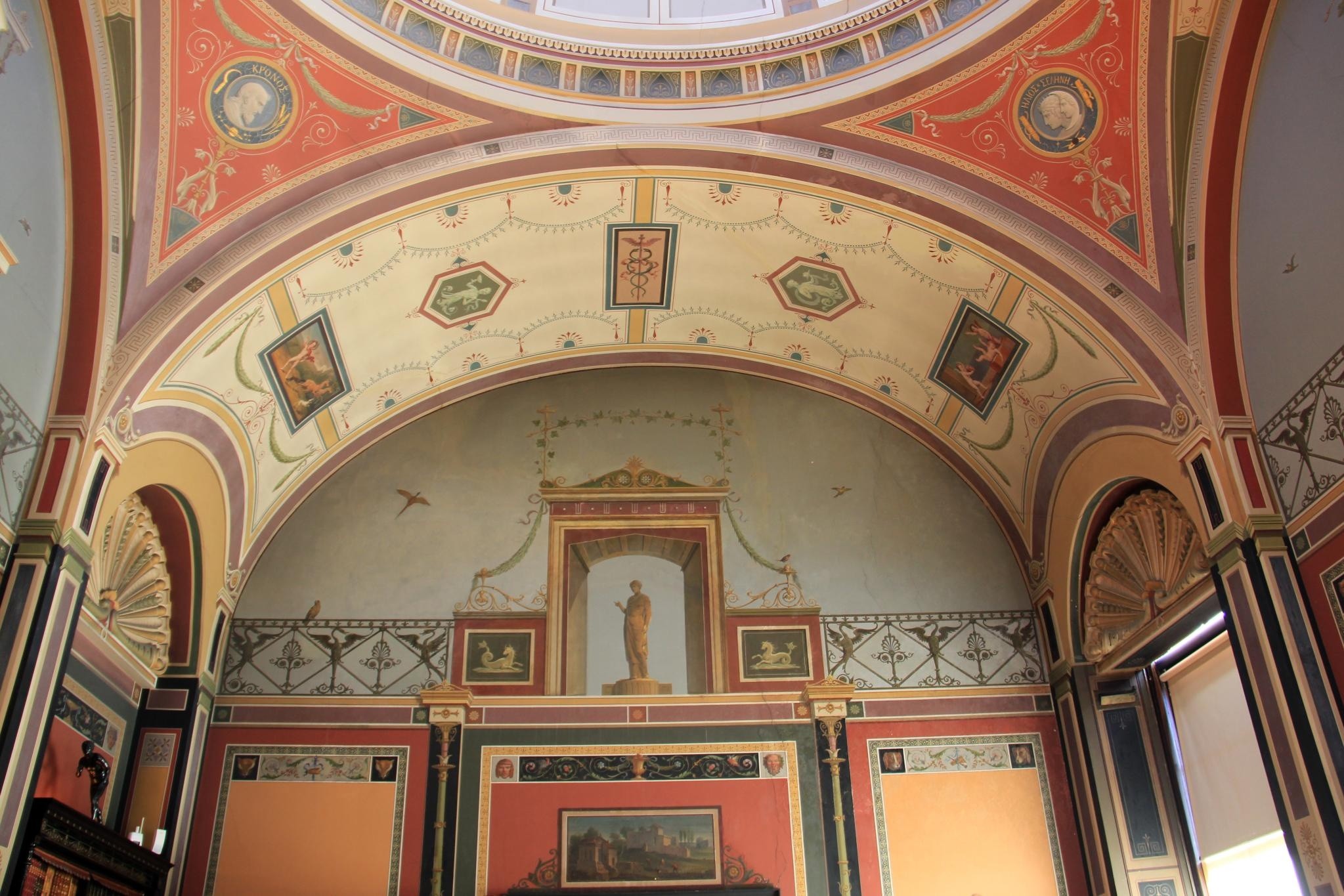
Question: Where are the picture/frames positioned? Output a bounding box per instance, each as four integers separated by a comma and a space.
461, 629, 534, 686
736, 625, 814, 683
557, 805, 725, 890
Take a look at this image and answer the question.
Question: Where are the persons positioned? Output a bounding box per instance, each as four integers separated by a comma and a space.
75, 740, 110, 822
1039, 91, 1082, 138
613, 580, 651, 678
226, 82, 267, 128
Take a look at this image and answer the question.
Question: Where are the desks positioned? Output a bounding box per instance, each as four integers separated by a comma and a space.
7, 796, 175, 896
